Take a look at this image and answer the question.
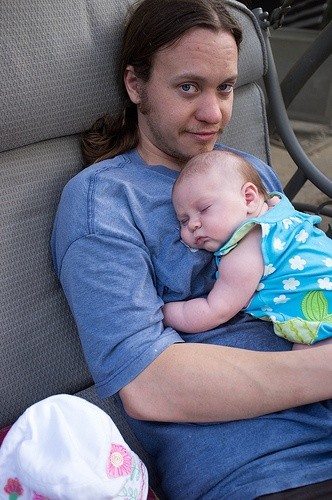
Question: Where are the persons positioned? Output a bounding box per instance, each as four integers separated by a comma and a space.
49, 0, 331, 499
162, 150, 332, 351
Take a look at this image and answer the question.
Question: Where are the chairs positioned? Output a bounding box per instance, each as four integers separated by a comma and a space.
1, 0, 332, 499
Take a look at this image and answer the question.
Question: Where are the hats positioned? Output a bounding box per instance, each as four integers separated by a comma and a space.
0, 394, 149, 499
